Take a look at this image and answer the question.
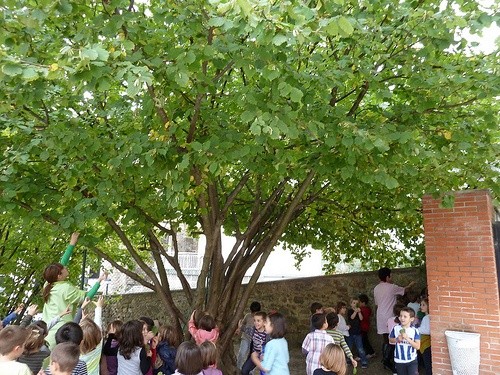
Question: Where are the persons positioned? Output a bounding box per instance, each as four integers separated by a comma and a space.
374, 267, 432, 375
302, 293, 373, 375
237, 302, 290, 375
0, 232, 223, 375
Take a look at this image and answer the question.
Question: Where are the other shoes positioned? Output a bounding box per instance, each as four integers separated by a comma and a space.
361, 361, 368, 368
